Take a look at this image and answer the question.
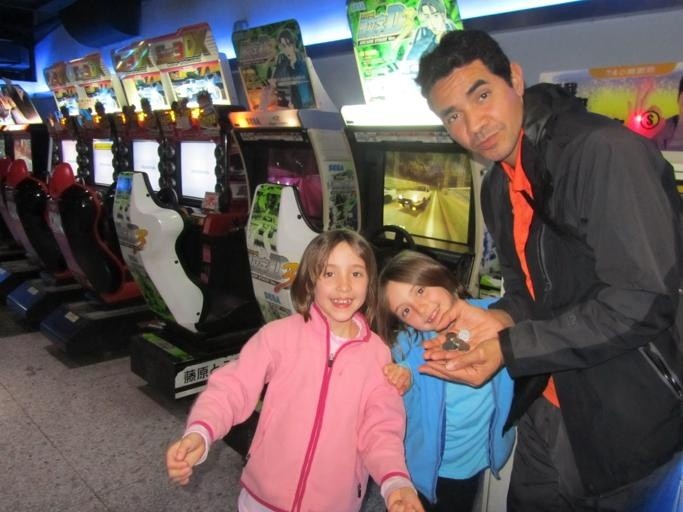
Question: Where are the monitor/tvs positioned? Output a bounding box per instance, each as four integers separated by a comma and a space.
230, 127, 324, 232
130, 138, 162, 193
179, 137, 222, 202
91, 136, 116, 186
60, 139, 80, 177
376, 139, 474, 263
0, 139, 6, 158
13, 137, 33, 174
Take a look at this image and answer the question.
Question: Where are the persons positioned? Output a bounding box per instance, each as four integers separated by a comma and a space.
377, 250, 516, 512
274, 30, 315, 108
166, 228, 425, 512
407, 0, 457, 62
418, 27, 683, 512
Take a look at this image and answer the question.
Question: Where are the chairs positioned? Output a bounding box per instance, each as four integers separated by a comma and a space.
47, 163, 142, 304
0, 158, 72, 284
243, 184, 323, 325
116, 170, 260, 334
